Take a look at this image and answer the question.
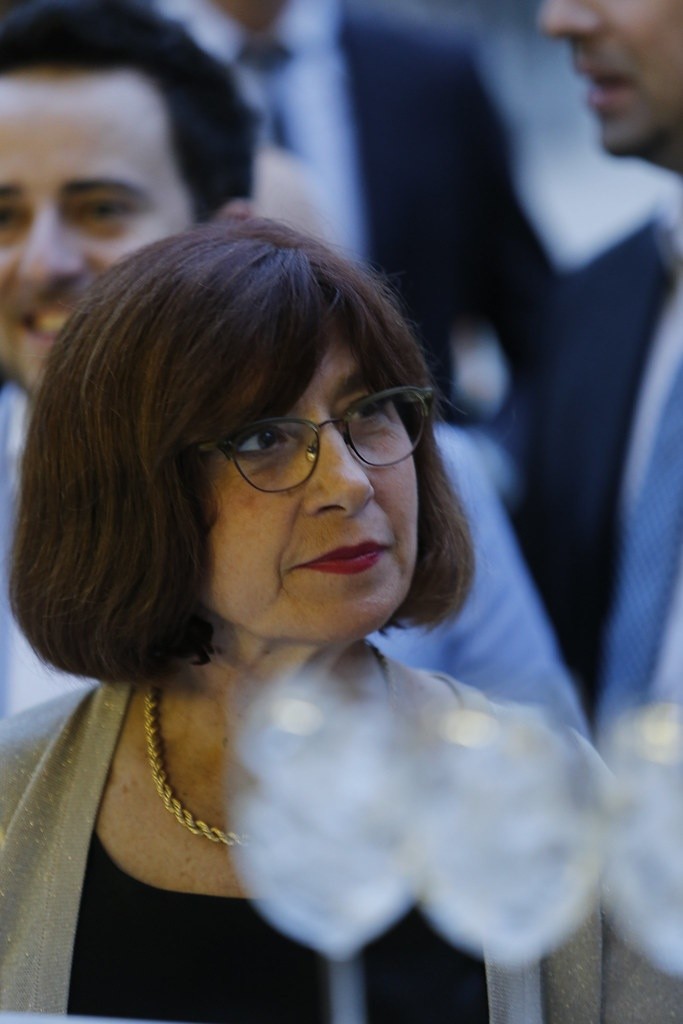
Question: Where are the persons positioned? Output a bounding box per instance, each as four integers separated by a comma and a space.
0, 217, 683, 1024
155, 0, 560, 426
491, 0, 683, 784
0, 0, 587, 734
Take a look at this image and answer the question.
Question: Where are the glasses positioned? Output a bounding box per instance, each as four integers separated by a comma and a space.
192, 387, 435, 493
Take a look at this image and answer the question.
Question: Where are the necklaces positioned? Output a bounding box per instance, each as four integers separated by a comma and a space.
144, 642, 401, 847
